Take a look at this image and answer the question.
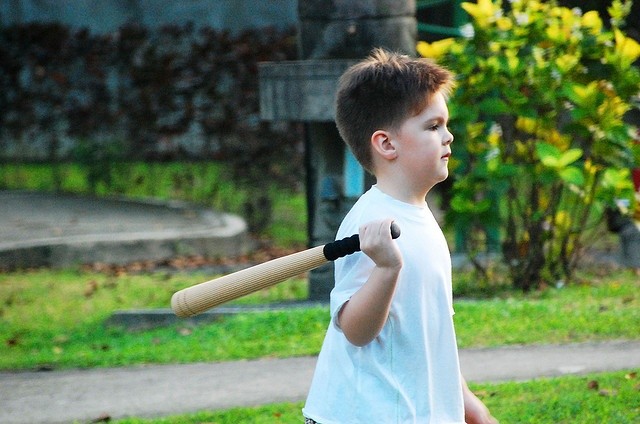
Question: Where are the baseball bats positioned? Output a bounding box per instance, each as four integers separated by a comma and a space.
172, 221, 401, 320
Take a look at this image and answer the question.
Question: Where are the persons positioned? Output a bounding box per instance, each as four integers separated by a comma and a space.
303, 46, 499, 424
